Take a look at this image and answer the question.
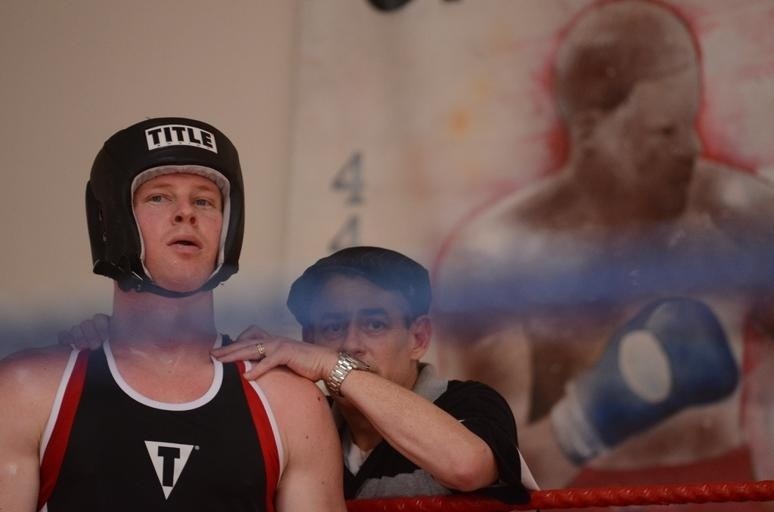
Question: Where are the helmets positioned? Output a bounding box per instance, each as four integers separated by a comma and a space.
84, 115, 246, 299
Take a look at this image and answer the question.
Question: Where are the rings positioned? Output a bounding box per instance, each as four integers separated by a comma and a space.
255, 343, 266, 358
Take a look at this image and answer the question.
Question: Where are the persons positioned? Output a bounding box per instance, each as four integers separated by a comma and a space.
428, 0, 774, 510
1, 117, 349, 512
57, 243, 529, 512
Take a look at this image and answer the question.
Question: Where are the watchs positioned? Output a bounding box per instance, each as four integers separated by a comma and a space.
326, 351, 373, 400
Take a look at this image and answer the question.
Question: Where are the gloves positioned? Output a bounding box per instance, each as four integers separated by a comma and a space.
548, 299, 738, 465
